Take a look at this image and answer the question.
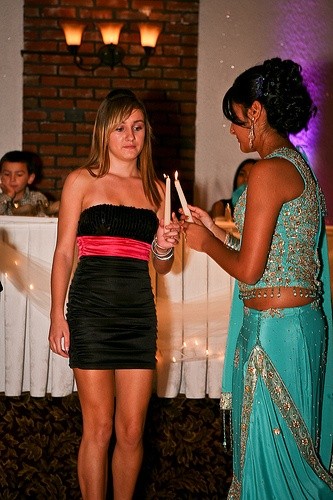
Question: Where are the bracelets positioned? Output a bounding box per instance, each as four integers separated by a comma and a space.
150, 233, 175, 262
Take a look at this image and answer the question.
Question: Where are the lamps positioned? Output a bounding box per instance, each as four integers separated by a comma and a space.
57, 19, 165, 73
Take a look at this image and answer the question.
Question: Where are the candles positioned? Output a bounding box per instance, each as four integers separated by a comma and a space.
163, 173, 172, 233
174, 170, 194, 223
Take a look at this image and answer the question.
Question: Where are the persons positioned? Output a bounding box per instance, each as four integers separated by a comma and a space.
211, 158, 261, 219
48, 87, 181, 500
178, 57, 333, 500
0, 150, 50, 217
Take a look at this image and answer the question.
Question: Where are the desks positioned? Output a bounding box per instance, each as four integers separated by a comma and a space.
0, 214, 235, 399
